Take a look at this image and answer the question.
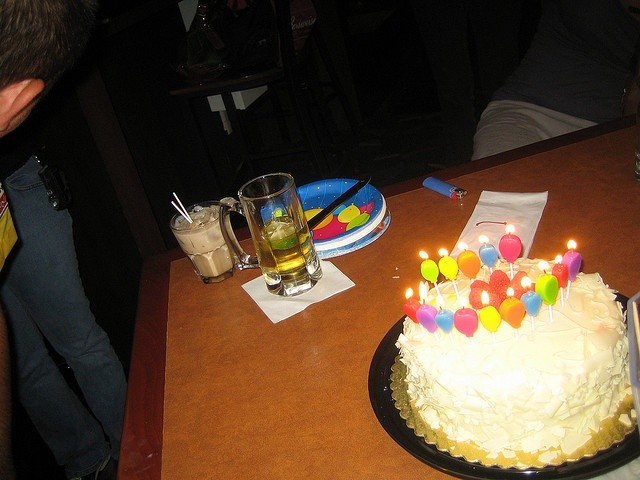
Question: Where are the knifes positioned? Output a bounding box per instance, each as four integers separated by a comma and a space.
298, 178, 372, 236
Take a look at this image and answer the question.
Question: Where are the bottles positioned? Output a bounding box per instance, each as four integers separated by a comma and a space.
179, 4, 227, 84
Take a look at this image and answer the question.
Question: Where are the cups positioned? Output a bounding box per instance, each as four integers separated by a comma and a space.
219, 172, 323, 297
168, 200, 235, 285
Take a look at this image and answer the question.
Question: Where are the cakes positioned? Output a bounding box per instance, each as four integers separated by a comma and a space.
395, 255, 637, 462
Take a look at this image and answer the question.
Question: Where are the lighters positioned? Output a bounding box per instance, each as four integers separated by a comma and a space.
422, 177, 468, 199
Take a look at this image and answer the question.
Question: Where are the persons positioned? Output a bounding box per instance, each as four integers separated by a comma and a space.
468, 0, 639, 162
1, 130, 128, 480
2, 1, 93, 138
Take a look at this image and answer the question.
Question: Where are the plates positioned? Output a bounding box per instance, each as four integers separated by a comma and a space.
368, 292, 640, 480
260, 178, 391, 260
